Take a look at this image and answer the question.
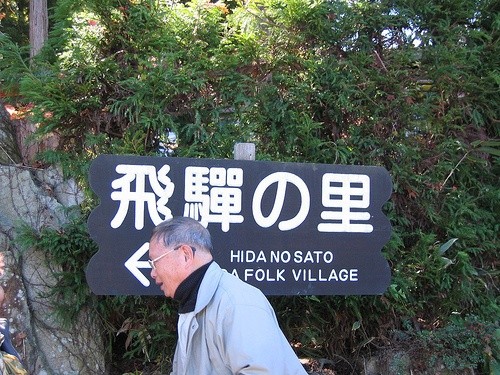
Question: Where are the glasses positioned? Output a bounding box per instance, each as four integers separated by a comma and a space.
148, 244, 197, 270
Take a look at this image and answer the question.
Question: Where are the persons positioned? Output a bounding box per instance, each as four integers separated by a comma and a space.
147, 216, 309, 375
